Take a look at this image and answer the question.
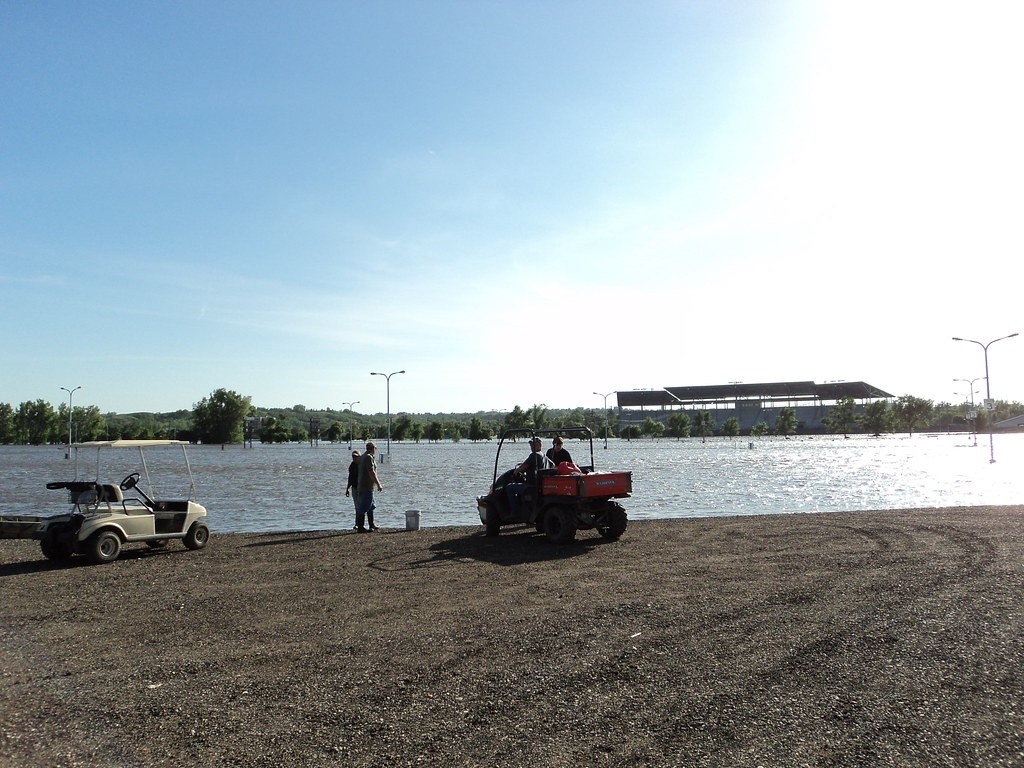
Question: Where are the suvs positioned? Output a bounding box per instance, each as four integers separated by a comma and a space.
476, 426, 633, 544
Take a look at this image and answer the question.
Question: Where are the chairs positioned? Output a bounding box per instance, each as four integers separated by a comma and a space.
522, 467, 557, 506
87, 484, 154, 516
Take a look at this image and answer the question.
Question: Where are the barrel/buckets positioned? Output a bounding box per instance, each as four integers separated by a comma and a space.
405, 510, 421, 531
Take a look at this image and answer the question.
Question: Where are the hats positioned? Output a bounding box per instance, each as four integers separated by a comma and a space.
529, 438, 542, 443
366, 442, 377, 449
352, 450, 361, 457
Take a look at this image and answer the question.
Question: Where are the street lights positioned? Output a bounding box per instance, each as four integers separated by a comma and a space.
342, 401, 360, 450
61, 386, 81, 459
370, 371, 406, 462
953, 333, 1019, 462
593, 391, 617, 449
953, 390, 981, 440
953, 377, 989, 446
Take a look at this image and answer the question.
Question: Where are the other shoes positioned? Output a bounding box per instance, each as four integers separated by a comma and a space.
366, 528, 374, 532
369, 523, 380, 529
357, 527, 369, 533
354, 526, 358, 530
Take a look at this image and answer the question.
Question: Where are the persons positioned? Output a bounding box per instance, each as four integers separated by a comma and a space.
344, 450, 381, 530
514, 437, 555, 491
354, 442, 383, 533
546, 436, 574, 469
